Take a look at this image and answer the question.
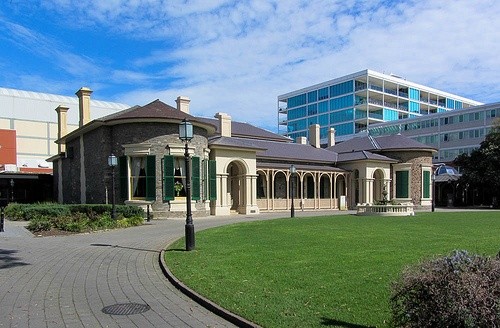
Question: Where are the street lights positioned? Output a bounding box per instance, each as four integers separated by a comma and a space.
108, 154, 117, 223
432, 174, 436, 213
290, 164, 295, 218
178, 118, 194, 251
